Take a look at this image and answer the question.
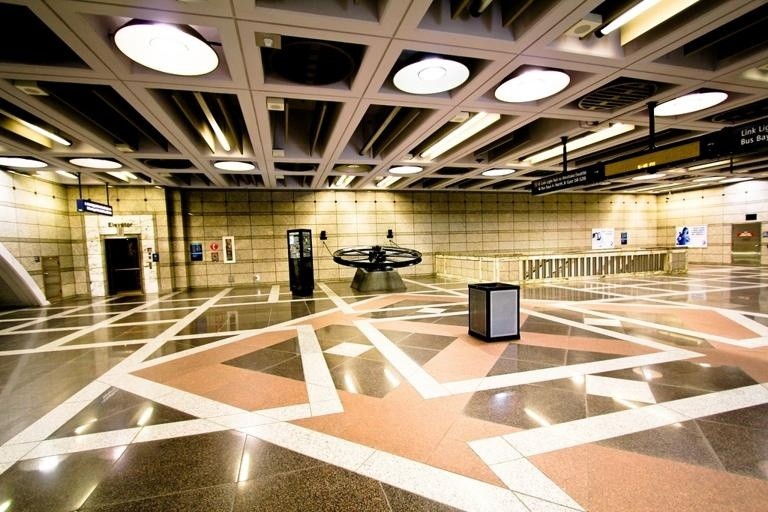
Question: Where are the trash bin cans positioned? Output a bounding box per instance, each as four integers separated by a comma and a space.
468, 282, 520, 342
